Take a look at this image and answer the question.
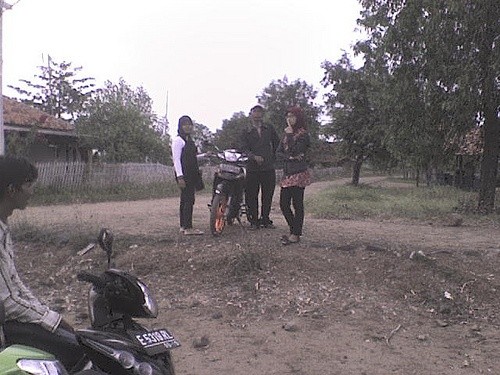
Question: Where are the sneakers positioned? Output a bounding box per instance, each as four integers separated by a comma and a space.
180, 227, 204, 235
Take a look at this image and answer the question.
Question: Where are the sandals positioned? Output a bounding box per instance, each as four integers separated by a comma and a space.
280, 234, 301, 245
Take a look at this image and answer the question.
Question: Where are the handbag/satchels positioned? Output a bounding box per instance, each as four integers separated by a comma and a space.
196, 176, 204, 191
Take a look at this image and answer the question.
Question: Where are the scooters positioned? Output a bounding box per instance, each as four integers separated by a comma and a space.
202, 134, 260, 237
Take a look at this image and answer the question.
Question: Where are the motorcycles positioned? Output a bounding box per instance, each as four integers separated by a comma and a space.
0, 228, 181, 375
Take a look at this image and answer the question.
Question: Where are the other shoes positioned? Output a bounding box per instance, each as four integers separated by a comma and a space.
249, 221, 260, 230
260, 220, 277, 229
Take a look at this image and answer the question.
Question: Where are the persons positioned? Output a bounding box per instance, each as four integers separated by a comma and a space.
235, 105, 280, 230
172, 116, 205, 235
0, 155, 108, 375
275, 108, 311, 245
